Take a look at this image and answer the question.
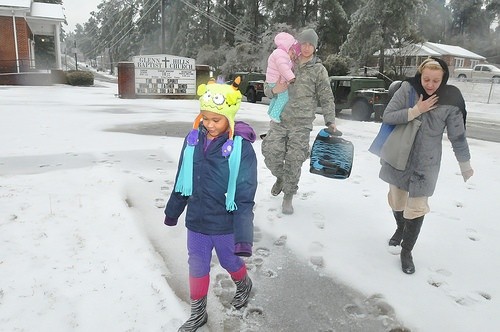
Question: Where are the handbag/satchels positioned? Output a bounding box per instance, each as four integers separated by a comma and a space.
369, 85, 422, 171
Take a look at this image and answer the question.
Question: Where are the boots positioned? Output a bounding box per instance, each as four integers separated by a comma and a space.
389, 211, 404, 246
232, 274, 252, 307
272, 179, 283, 196
401, 215, 425, 274
282, 194, 294, 214
178, 296, 207, 332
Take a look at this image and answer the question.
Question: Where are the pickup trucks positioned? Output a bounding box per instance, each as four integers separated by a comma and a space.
454, 64, 500, 85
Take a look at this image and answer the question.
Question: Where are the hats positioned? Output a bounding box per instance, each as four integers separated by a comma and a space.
187, 77, 241, 157
298, 29, 318, 49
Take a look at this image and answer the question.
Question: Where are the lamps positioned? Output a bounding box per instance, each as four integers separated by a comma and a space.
41, 36, 45, 42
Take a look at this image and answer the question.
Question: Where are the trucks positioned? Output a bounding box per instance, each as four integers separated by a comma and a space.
327, 75, 389, 122
233, 71, 265, 103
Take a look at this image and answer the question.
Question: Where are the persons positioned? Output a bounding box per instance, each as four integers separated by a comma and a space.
367, 55, 474, 274
163, 77, 257, 332
261, 28, 337, 214
265, 32, 302, 124
